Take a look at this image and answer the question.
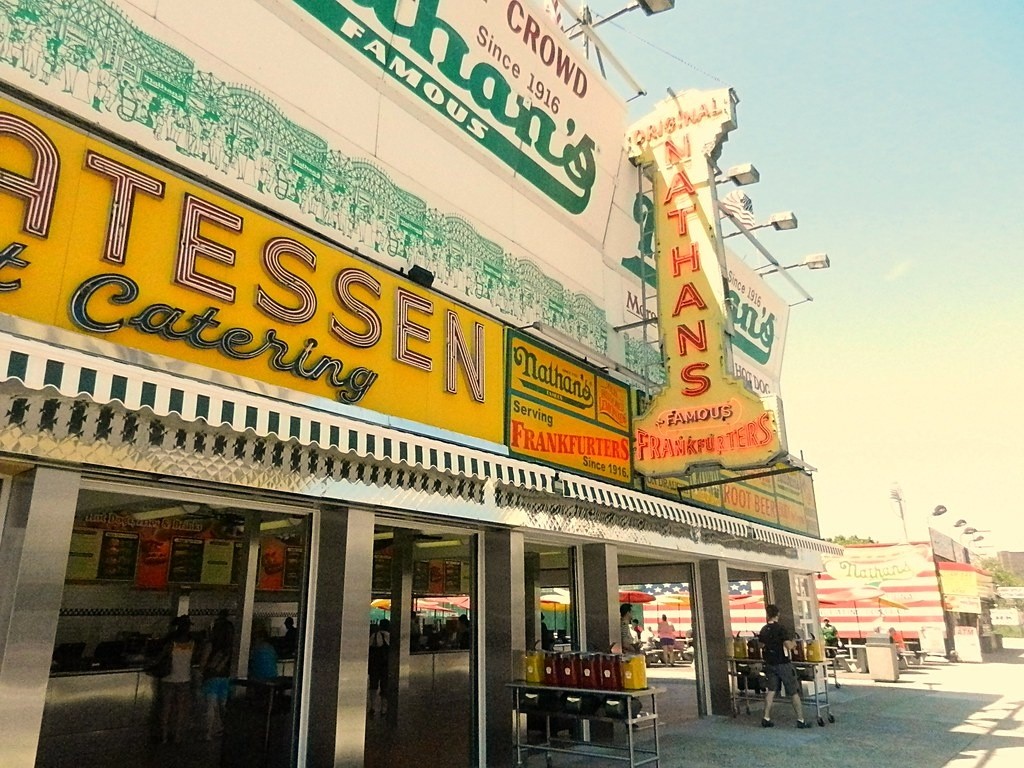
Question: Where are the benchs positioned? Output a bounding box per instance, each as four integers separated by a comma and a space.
825, 649, 929, 672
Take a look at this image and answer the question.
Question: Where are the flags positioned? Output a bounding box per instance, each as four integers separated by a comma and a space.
721, 191, 755, 227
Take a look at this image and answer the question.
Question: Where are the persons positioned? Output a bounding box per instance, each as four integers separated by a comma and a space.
632, 618, 643, 639
620, 603, 636, 653
249, 631, 278, 679
368, 613, 470, 716
199, 609, 235, 741
822, 618, 842, 668
889, 628, 904, 649
279, 617, 297, 655
540, 612, 555, 651
641, 626, 655, 646
158, 614, 198, 746
658, 614, 676, 667
759, 604, 811, 728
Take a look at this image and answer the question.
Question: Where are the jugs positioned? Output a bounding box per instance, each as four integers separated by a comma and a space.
733, 637, 747, 658
807, 639, 825, 662
525, 651, 647, 690
791, 639, 805, 662
748, 638, 764, 660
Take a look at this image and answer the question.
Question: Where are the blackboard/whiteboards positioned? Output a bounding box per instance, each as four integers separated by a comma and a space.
918, 626, 948, 656
954, 626, 983, 663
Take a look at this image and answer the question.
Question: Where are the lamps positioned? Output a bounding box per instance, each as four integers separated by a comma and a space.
517, 322, 662, 394
407, 265, 434, 286
927, 505, 947, 526
953, 519, 966, 528
967, 536, 984, 548
714, 165, 760, 187
786, 452, 818, 474
760, 254, 831, 276
567, 0, 675, 41
724, 212, 797, 239
959, 528, 978, 543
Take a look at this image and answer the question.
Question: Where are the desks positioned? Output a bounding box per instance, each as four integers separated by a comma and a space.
845, 644, 869, 673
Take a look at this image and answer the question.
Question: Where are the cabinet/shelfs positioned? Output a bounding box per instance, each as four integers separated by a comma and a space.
727, 656, 837, 728
505, 681, 660, 768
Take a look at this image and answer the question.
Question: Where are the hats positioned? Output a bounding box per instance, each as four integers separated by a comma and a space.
823, 618, 830, 622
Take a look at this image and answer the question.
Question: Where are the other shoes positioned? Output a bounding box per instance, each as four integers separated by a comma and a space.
762, 718, 774, 727
797, 719, 810, 728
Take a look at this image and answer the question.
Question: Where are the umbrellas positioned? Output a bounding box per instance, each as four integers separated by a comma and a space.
619, 590, 656, 604
540, 594, 570, 632
371, 594, 470, 618
651, 592, 910, 639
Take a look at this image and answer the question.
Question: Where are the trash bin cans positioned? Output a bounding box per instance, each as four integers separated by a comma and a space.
865, 634, 900, 682
980, 636, 993, 653
995, 634, 1003, 648
